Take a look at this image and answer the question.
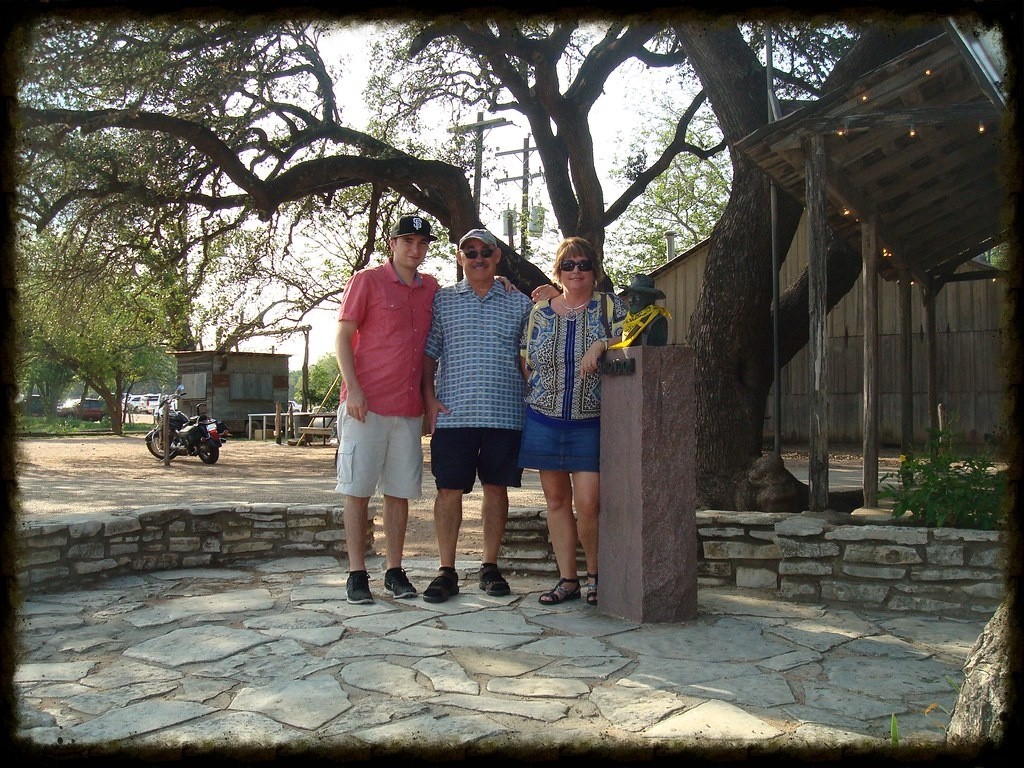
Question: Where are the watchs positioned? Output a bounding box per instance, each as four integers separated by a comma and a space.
601, 338, 608, 351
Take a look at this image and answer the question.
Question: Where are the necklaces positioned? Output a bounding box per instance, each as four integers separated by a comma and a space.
561, 301, 588, 321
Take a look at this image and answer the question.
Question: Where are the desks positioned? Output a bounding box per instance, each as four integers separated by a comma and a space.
309, 414, 337, 445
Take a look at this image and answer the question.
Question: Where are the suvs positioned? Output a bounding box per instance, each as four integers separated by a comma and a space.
59, 398, 107, 423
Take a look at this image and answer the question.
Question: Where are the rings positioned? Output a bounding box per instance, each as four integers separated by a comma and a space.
587, 365, 592, 366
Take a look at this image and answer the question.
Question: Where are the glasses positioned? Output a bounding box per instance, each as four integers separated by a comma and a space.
459, 248, 496, 259
559, 260, 593, 271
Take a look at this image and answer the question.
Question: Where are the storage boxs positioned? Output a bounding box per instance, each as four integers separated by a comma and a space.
255, 429, 274, 440
180, 426, 201, 441
217, 420, 230, 434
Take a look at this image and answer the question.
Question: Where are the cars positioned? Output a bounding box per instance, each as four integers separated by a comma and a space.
111, 393, 179, 414
287, 401, 338, 439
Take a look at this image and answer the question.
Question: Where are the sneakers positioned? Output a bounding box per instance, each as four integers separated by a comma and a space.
384, 568, 417, 599
345, 572, 373, 604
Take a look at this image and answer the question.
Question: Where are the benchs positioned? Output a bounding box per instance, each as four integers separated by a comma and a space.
298, 427, 332, 447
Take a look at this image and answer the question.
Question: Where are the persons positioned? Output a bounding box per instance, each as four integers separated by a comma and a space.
517, 237, 630, 604
620, 274, 668, 346
334, 214, 518, 603
421, 229, 560, 603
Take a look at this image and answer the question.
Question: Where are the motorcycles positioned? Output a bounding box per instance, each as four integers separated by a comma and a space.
144, 385, 230, 463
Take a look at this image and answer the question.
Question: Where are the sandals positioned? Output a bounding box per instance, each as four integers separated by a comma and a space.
538, 577, 581, 605
479, 566, 510, 596
586, 572, 598, 606
424, 571, 460, 603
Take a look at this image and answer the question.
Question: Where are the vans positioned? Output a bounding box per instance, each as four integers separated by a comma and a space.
16, 394, 45, 416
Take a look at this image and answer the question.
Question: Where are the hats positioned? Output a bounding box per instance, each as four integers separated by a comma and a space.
391, 215, 438, 241
458, 228, 497, 249
